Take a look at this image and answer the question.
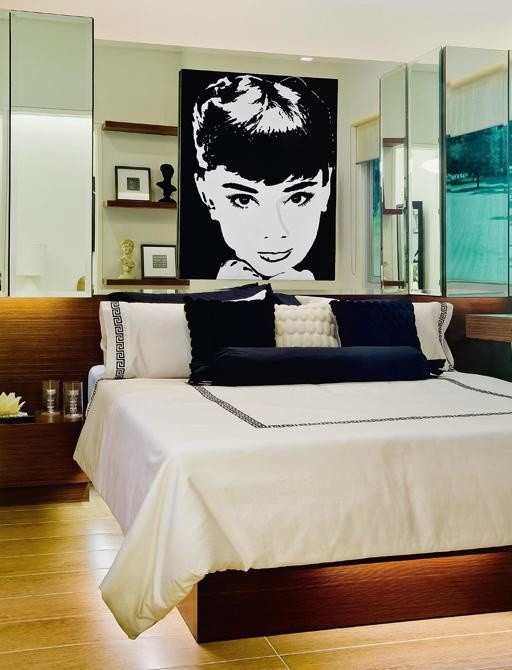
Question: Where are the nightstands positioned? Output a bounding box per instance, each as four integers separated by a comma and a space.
0, 411, 92, 505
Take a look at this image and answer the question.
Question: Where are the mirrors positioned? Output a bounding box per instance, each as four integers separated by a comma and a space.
442, 45, 511, 294
11, 10, 93, 296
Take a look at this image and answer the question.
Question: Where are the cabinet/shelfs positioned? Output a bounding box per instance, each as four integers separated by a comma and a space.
101, 119, 190, 287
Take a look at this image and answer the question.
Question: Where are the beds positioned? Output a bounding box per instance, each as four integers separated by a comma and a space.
72, 363, 512, 644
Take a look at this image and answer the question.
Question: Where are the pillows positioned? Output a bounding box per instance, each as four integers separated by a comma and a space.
274, 303, 338, 347
183, 292, 276, 371
191, 282, 273, 302
190, 346, 446, 387
328, 299, 421, 349
99, 301, 193, 379
294, 295, 454, 372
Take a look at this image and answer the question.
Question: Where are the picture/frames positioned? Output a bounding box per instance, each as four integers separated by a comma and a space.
115, 166, 151, 201
141, 244, 178, 279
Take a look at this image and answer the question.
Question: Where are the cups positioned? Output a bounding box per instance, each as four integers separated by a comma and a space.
63, 381, 84, 420
40, 379, 61, 416
